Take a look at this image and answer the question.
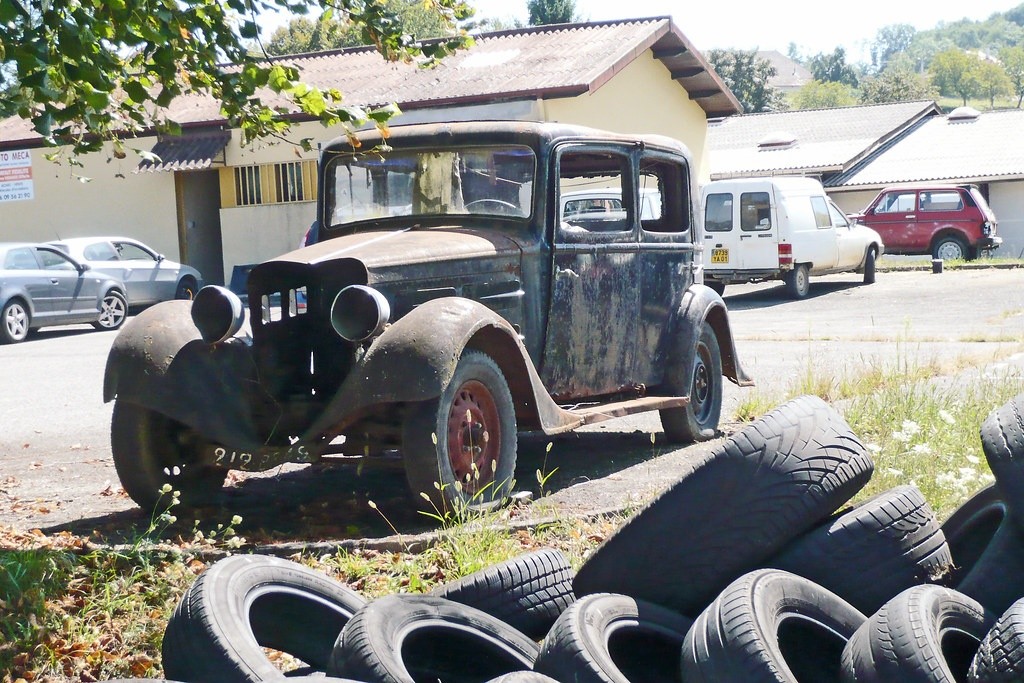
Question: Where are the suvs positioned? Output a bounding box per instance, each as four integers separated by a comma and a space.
842, 181, 1002, 267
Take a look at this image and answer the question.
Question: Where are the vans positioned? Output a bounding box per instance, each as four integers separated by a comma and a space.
696, 173, 886, 301
557, 184, 667, 225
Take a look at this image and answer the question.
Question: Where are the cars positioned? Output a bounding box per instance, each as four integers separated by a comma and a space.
46, 235, 203, 308
102, 121, 758, 526
0, 241, 128, 344
